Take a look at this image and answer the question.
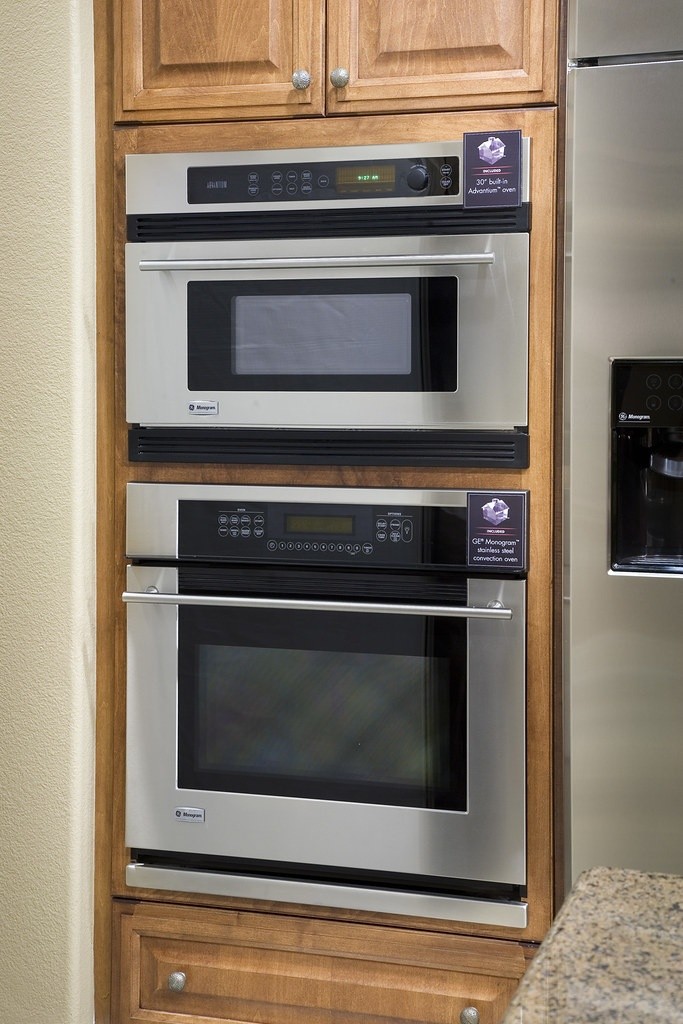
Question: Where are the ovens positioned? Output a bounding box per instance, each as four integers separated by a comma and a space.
122, 138, 528, 470
125, 480, 530, 933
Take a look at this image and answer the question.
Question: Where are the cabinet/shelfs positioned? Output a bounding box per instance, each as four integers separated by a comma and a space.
110, 0, 559, 124
109, 896, 539, 1024
